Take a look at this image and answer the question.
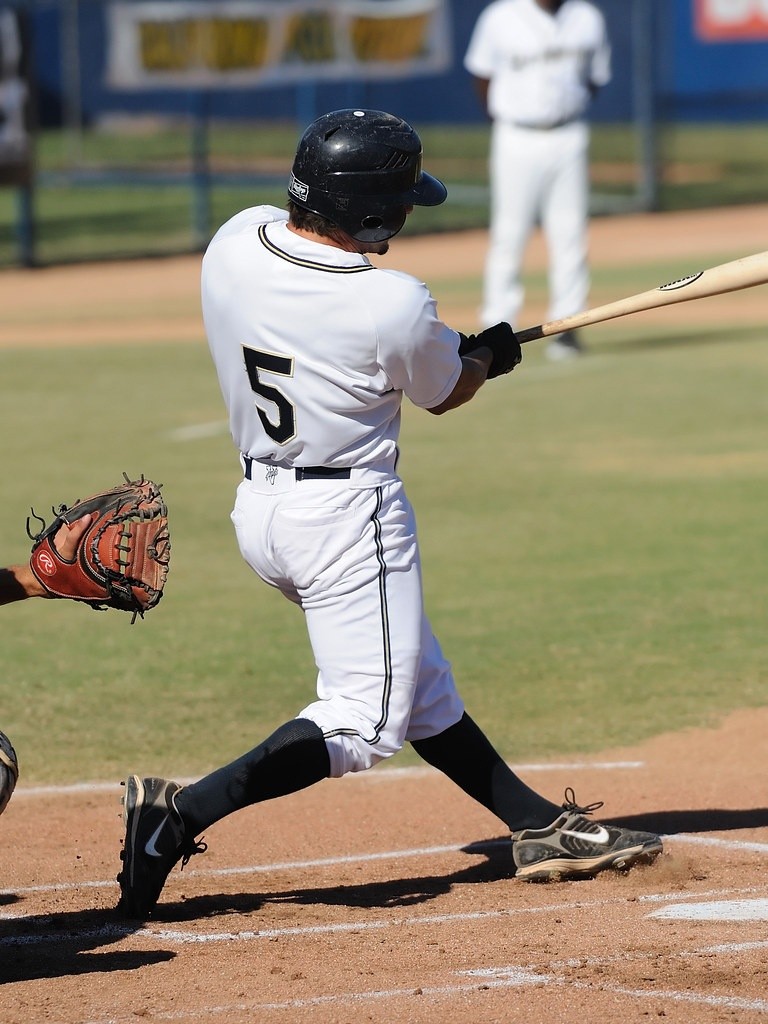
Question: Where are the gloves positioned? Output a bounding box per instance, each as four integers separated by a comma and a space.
458, 320, 522, 379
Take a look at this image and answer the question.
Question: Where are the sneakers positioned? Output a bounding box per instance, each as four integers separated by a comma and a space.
510, 787, 665, 882
117, 774, 209, 913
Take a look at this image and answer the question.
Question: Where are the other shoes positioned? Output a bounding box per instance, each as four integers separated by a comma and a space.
545, 336, 576, 361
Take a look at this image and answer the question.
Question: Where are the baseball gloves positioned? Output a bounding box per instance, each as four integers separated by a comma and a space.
26, 472, 181, 618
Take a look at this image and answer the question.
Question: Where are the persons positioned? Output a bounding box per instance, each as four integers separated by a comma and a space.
0, 513, 93, 817
116, 109, 664, 919
464, 0, 612, 362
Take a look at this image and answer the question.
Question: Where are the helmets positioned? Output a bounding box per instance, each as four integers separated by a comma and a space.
287, 110, 448, 243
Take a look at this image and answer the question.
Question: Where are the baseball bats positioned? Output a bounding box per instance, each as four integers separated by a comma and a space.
508, 228, 767, 351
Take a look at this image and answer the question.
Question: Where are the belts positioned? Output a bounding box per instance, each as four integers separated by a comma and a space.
244, 457, 350, 481
